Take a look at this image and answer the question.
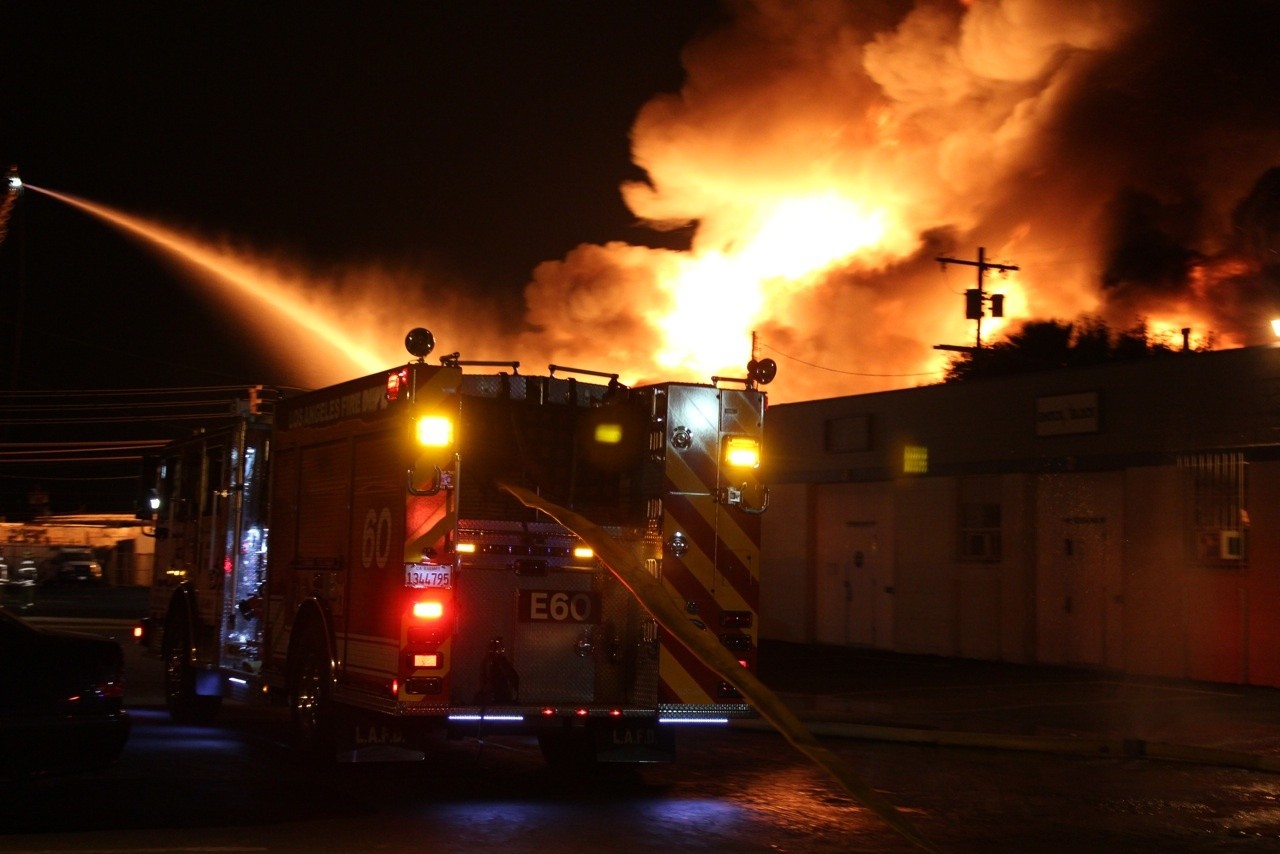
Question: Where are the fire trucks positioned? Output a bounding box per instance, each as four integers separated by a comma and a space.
135, 328, 777, 795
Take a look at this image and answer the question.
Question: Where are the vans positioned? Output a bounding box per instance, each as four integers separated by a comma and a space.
49, 547, 103, 589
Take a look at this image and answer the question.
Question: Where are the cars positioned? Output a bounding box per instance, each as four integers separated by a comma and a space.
0, 609, 123, 709
